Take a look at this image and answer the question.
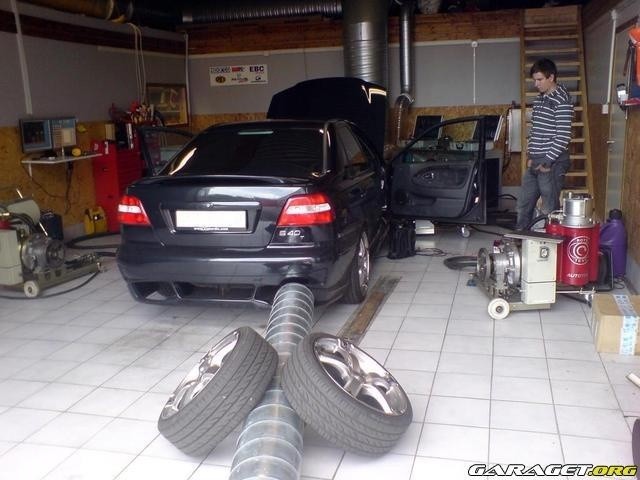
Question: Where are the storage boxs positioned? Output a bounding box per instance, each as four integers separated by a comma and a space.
591, 293, 640, 353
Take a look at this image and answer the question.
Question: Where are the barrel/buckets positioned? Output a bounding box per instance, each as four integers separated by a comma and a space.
84, 206, 107, 235
598, 209, 627, 275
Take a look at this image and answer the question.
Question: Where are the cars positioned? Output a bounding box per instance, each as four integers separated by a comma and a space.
116, 76, 489, 303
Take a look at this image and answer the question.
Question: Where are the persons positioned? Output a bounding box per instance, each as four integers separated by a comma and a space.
513, 55, 577, 231
